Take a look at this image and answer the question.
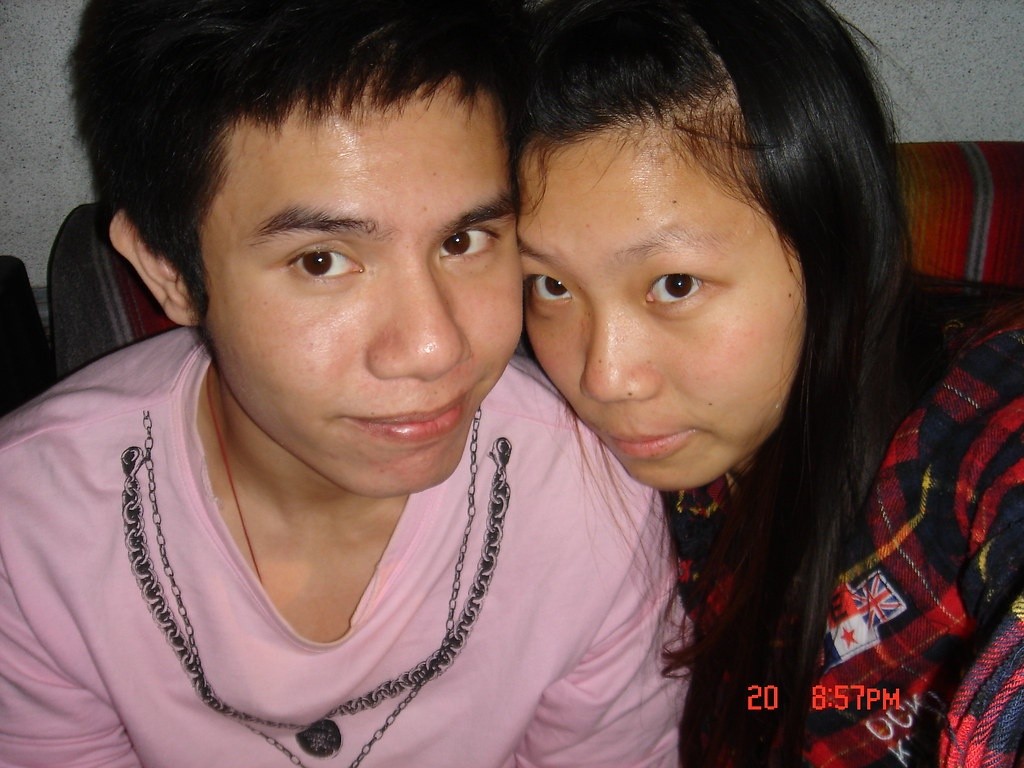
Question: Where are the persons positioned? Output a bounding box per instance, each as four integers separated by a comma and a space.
509, 0, 1023, 768
0, 0, 693, 768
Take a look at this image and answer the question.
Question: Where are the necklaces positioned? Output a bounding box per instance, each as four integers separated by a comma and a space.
206, 366, 262, 584
